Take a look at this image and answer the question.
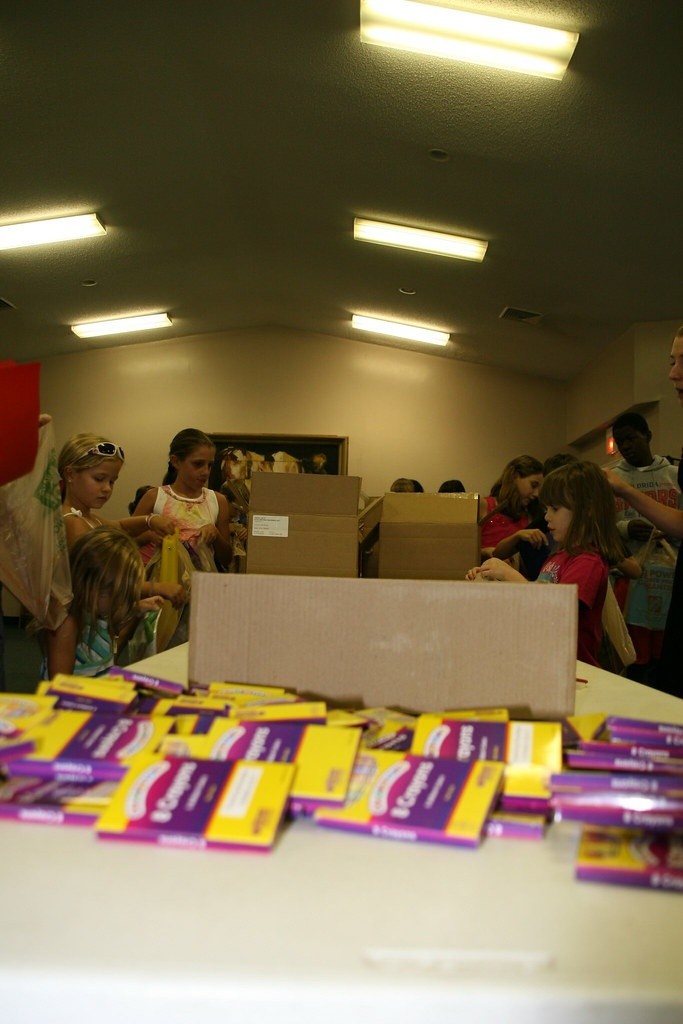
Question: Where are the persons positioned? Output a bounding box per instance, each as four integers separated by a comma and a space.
55, 433, 188, 610
47, 524, 166, 681
601, 325, 683, 702
126, 427, 231, 649
463, 460, 624, 672
602, 412, 683, 684
480, 454, 544, 562
492, 455, 577, 582
390, 477, 425, 493
438, 480, 467, 492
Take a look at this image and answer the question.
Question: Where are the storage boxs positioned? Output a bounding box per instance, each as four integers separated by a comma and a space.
186, 571, 578, 721
375, 491, 484, 582
245, 471, 366, 576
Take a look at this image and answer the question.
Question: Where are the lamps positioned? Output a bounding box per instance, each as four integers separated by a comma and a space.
353, 217, 489, 263
0, 213, 108, 251
351, 314, 450, 347
70, 312, 173, 339
360, 0, 580, 81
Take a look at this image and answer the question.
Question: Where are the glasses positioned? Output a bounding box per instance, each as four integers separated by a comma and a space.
70, 442, 126, 462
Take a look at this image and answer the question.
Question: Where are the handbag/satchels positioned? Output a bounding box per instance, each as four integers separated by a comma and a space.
0, 415, 74, 621
601, 578, 637, 674
621, 526, 676, 630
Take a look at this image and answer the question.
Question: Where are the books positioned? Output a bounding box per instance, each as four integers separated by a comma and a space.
0, 668, 683, 892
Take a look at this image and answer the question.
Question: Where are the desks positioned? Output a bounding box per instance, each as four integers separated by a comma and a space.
0, 642, 683, 1024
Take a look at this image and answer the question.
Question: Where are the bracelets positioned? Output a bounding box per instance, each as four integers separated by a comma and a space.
145, 513, 160, 529
